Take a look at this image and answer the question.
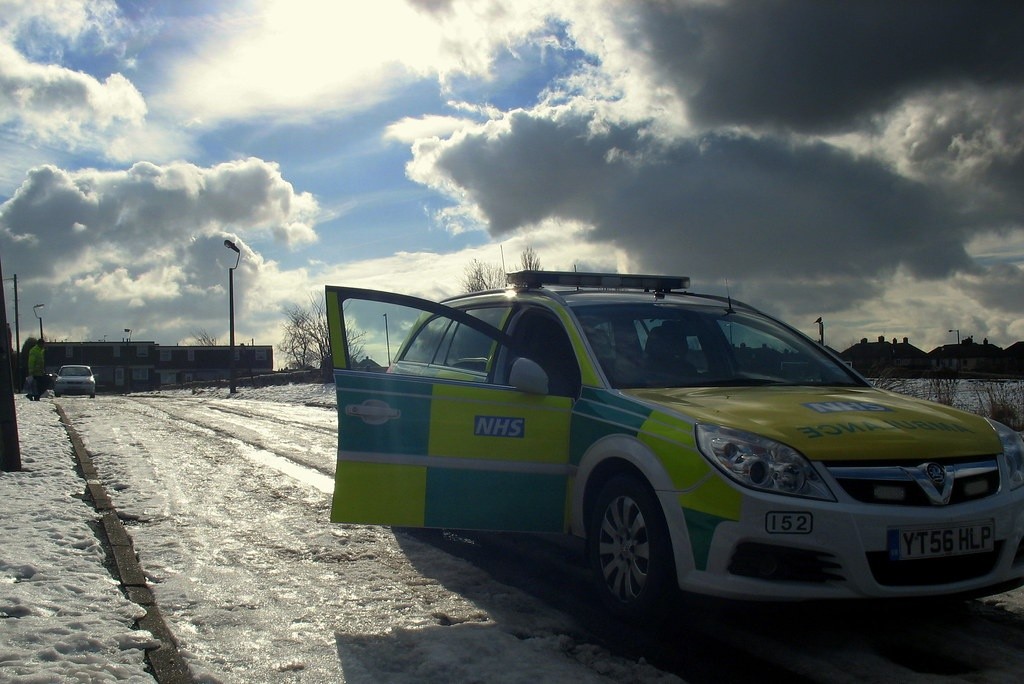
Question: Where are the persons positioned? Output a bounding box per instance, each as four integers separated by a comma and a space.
25, 338, 48, 401
634, 322, 710, 383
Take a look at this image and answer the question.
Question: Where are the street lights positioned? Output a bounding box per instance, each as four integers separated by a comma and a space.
383, 313, 391, 367
32, 304, 45, 341
949, 330, 960, 346
223, 238, 241, 396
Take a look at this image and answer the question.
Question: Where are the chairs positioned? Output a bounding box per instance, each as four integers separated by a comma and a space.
645, 326, 689, 357
563, 328, 612, 358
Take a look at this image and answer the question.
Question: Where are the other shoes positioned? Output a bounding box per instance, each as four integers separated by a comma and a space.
26, 395, 33, 401
34, 397, 40, 401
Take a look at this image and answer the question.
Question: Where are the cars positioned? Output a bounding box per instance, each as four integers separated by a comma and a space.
325, 269, 1023, 632
52, 365, 100, 398
